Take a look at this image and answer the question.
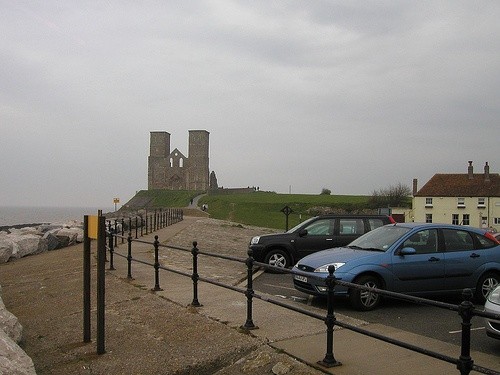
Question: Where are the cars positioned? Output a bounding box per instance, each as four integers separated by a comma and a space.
483, 285, 500, 340
489, 231, 500, 242
292, 222, 500, 312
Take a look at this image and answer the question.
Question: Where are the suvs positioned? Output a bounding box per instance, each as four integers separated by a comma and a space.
246, 214, 397, 275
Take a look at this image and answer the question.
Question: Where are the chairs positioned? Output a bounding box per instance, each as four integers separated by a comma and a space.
405, 234, 421, 253
444, 232, 464, 251
464, 234, 475, 251
421, 236, 436, 253
340, 224, 344, 234
